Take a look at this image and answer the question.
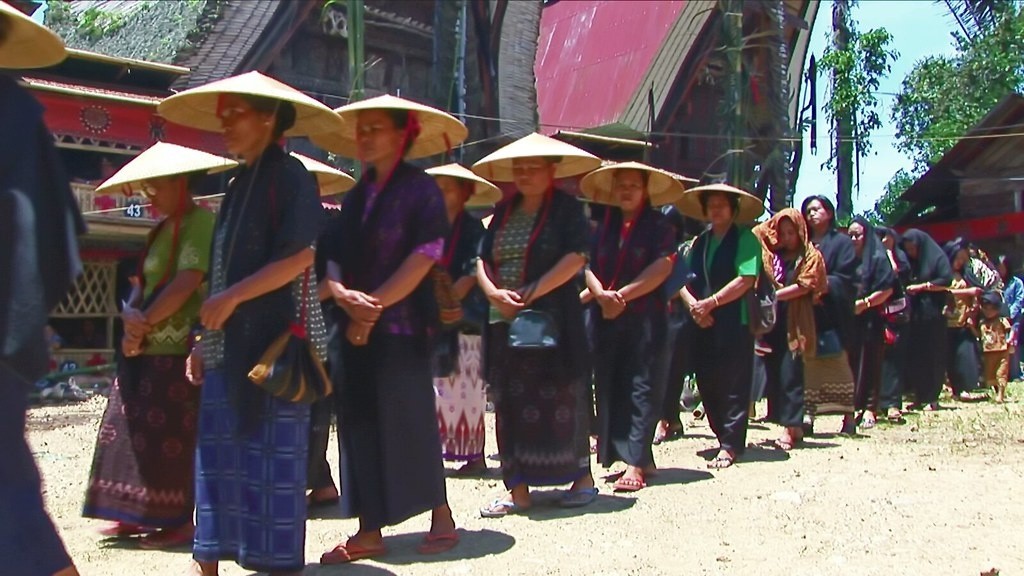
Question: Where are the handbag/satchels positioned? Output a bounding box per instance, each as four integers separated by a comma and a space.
434, 268, 463, 324
247, 322, 333, 403
507, 309, 560, 350
744, 261, 778, 337
655, 255, 697, 308
814, 326, 841, 357
884, 296, 911, 327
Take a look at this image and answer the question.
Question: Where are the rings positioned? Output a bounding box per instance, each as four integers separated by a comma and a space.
357, 336, 361, 341
130, 350, 136, 354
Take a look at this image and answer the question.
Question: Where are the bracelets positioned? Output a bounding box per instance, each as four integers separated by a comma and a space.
712, 294, 720, 308
927, 281, 931, 292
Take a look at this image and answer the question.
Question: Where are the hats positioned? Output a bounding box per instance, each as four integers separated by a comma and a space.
96, 70, 765, 224
0, 1, 66, 68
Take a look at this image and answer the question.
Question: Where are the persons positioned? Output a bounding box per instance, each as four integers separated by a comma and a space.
81, 141, 238, 549
207, 94, 1024, 564
315, 90, 468, 566
0, 0, 86, 575
43, 325, 66, 351
156, 70, 344, 576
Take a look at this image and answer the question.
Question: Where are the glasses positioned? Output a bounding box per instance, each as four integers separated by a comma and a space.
847, 228, 868, 239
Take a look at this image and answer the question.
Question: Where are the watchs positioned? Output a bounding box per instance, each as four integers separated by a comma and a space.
863, 296, 871, 309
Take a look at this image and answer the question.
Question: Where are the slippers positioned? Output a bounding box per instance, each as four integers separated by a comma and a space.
772, 434, 805, 451
886, 410, 902, 420
612, 475, 647, 490
458, 464, 488, 476
101, 522, 155, 534
320, 539, 388, 564
419, 531, 461, 553
479, 497, 525, 517
859, 418, 876, 428
306, 492, 341, 508
707, 452, 738, 469
561, 488, 600, 507
138, 528, 194, 549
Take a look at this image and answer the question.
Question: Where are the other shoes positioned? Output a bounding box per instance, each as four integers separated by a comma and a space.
652, 427, 684, 445
